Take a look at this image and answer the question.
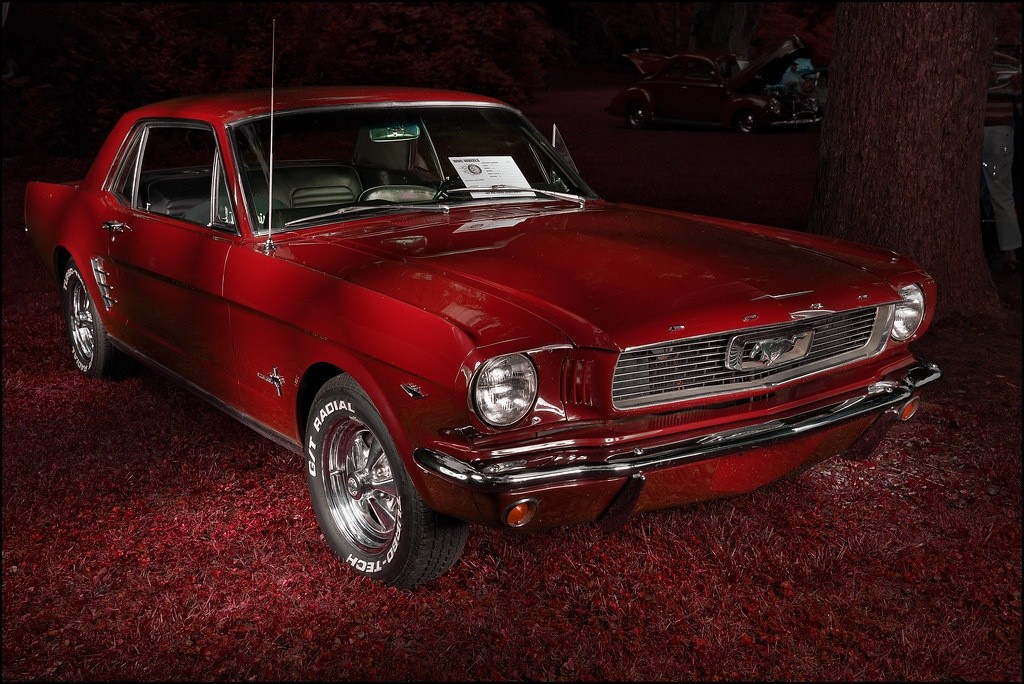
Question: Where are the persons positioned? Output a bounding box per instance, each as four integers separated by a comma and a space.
981, 50, 1023, 268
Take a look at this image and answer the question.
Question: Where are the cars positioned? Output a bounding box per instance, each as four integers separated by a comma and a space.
980, 52, 1024, 126
19, 82, 942, 592
601, 33, 825, 134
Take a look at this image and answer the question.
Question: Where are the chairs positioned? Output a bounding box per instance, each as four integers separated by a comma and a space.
350, 126, 409, 184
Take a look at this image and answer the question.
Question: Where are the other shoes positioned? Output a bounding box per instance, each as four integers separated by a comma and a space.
1002, 262, 1016, 275
